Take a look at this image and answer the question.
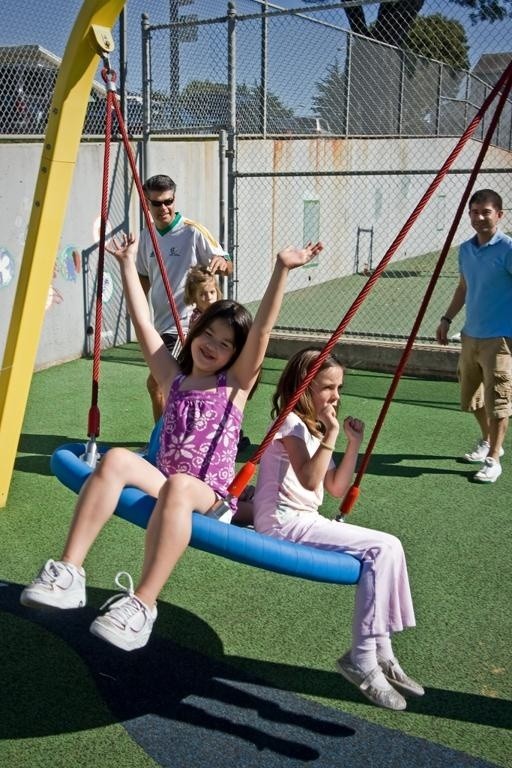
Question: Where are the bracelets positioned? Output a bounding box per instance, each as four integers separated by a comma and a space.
320, 439, 335, 451
440, 314, 451, 324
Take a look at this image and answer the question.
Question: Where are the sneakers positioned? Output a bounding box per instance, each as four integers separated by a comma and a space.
90, 572, 157, 651
473, 457, 502, 483
20, 558, 86, 609
464, 440, 505, 462
379, 658, 425, 696
336, 649, 407, 711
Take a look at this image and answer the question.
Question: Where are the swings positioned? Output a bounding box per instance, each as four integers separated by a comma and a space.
52, 49, 512, 586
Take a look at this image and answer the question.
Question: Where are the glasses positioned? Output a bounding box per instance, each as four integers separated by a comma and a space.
148, 197, 174, 207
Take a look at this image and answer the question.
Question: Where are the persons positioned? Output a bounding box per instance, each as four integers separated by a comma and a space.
183, 265, 228, 331
248, 346, 431, 714
434, 184, 511, 484
121, 174, 234, 424
15, 230, 327, 650
